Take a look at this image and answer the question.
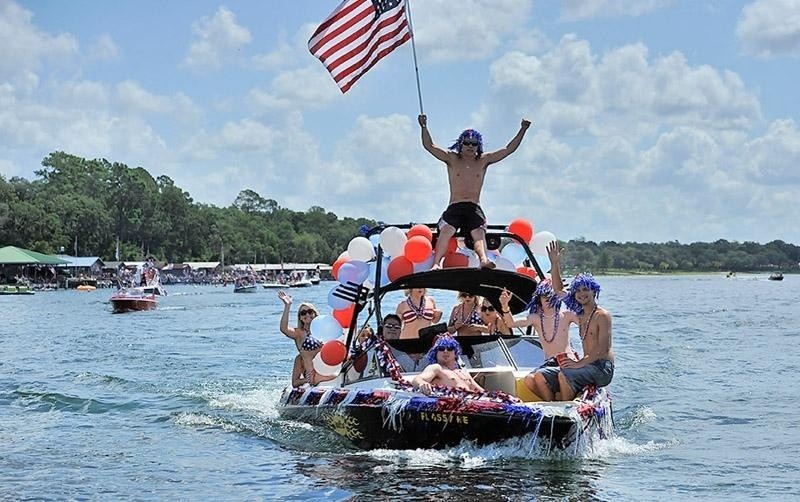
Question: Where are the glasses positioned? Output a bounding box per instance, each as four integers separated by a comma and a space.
462, 141, 478, 146
300, 309, 313, 316
461, 293, 474, 298
481, 305, 495, 312
436, 346, 454, 351
384, 324, 401, 329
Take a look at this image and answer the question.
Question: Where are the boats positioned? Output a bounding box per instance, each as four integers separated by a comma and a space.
77, 284, 96, 290
110, 293, 159, 311
289, 271, 311, 287
0, 285, 35, 295
310, 277, 320, 285
278, 224, 612, 451
234, 284, 256, 293
262, 283, 290, 288
117, 266, 168, 297
767, 272, 783, 280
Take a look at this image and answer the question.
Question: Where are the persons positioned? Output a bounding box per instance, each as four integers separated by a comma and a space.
416, 112, 533, 269
413, 335, 485, 394
1, 259, 322, 287
396, 288, 443, 339
446, 291, 490, 336
291, 353, 308, 388
498, 279, 580, 401
535, 242, 614, 401
357, 321, 374, 344
480, 296, 513, 336
276, 287, 337, 387
381, 313, 402, 339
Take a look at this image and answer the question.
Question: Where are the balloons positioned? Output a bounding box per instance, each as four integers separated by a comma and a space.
329, 221, 556, 282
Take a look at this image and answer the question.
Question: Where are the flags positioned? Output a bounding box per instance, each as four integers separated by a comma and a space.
305, 1, 413, 94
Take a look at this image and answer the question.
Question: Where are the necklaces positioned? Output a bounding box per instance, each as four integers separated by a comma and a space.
538, 311, 559, 342
577, 304, 598, 342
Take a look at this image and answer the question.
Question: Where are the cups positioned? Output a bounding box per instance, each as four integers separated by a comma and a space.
554, 352, 567, 369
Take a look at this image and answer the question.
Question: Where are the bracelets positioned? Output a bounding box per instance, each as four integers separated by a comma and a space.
502, 307, 510, 314
